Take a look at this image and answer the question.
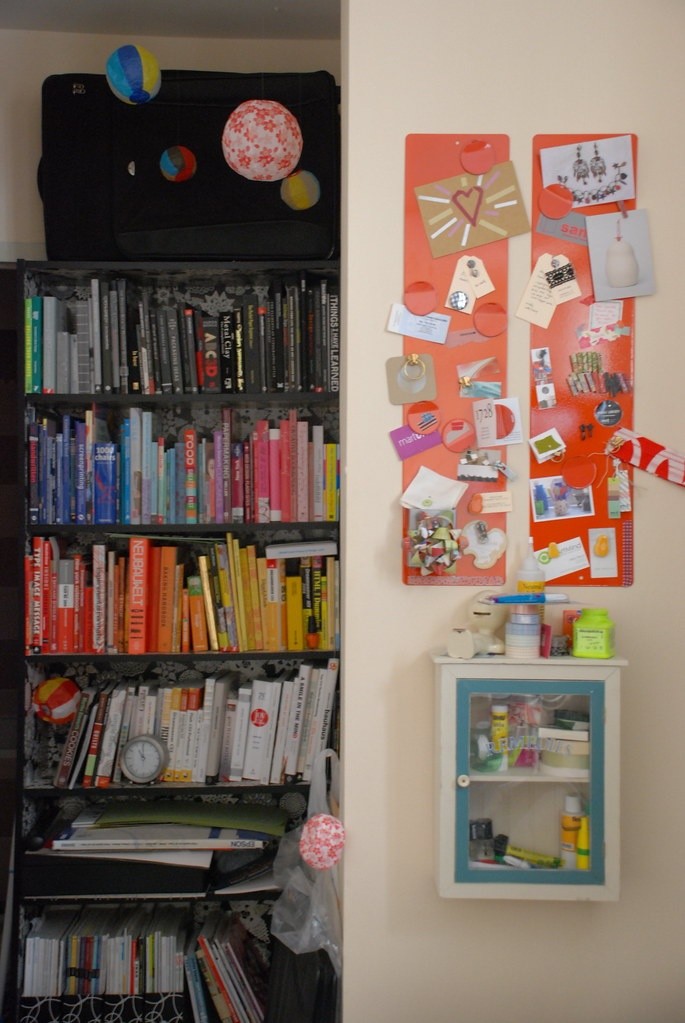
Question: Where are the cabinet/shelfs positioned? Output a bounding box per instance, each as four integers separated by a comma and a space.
0, 259, 340, 1023
428, 653, 629, 901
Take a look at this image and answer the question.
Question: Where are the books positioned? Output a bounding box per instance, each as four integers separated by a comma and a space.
538, 709, 589, 741
21, 273, 341, 852
22, 903, 267, 1022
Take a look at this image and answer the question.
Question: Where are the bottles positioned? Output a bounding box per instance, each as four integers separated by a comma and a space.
489, 704, 510, 773
560, 795, 586, 869
511, 537, 546, 623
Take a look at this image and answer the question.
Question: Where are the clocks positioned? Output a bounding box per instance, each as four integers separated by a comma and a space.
120, 734, 169, 783
